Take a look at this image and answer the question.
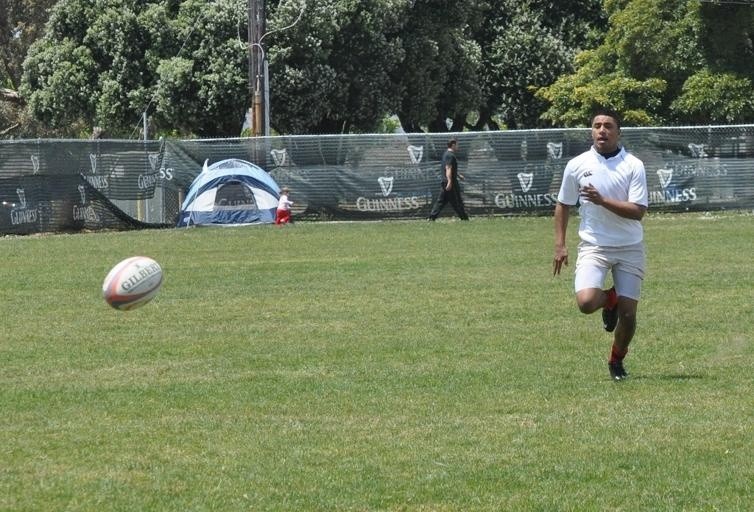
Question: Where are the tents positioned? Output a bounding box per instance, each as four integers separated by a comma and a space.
176, 158, 282, 227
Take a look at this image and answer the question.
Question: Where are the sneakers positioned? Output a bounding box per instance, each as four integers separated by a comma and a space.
602, 286, 619, 333
608, 359, 628, 382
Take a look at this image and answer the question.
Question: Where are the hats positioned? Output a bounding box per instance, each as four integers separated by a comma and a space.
279, 188, 290, 194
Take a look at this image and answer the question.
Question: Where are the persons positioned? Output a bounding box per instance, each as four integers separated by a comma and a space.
275, 187, 295, 225
427, 138, 470, 221
552, 109, 649, 381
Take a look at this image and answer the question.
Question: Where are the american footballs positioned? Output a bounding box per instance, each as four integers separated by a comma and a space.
102, 256, 162, 310
